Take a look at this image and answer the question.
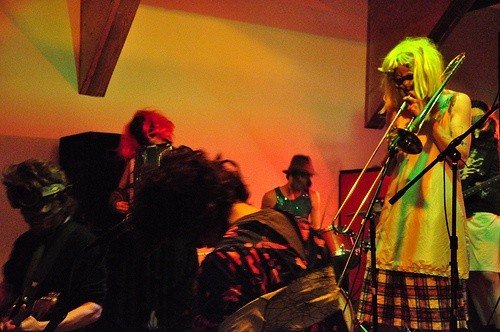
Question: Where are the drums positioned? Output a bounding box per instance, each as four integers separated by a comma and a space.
316, 225, 361, 272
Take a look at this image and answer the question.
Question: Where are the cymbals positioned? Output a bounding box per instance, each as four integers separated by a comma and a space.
343, 207, 383, 216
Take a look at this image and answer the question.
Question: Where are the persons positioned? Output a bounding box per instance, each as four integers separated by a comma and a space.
138, 146, 352, 332
262, 154, 321, 231
352, 35, 475, 332
108, 110, 200, 331
459, 98, 500, 332
0, 156, 112, 332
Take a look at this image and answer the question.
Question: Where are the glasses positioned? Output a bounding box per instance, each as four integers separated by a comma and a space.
396, 74, 413, 85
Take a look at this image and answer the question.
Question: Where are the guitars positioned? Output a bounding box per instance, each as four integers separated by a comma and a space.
0, 290, 63, 332
462, 173, 500, 198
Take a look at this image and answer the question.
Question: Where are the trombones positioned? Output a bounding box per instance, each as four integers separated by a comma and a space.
331, 52, 467, 234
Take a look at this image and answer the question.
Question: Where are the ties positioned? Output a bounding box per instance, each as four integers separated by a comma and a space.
20, 231, 55, 300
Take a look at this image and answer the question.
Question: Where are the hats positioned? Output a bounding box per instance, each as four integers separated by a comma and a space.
283, 156, 316, 175
